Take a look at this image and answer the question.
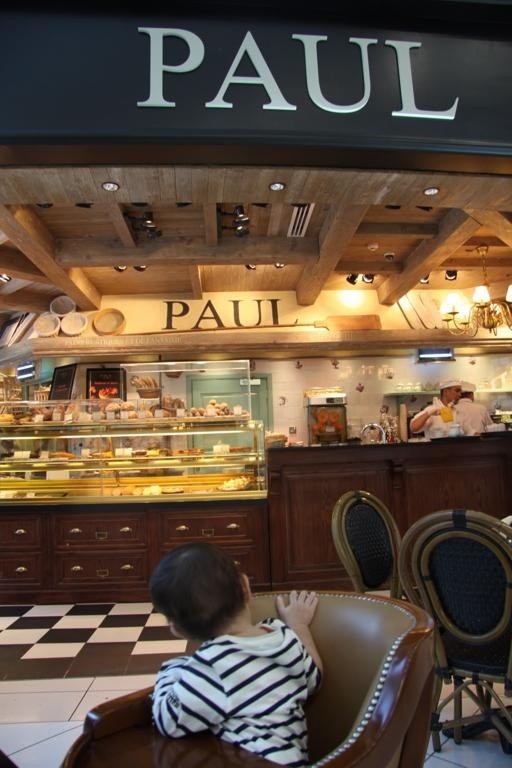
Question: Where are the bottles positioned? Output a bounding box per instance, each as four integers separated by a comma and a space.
287, 427, 297, 444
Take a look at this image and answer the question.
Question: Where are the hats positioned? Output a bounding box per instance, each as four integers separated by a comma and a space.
440, 379, 477, 392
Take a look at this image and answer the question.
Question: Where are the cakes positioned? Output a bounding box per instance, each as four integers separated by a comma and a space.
0, 397, 254, 497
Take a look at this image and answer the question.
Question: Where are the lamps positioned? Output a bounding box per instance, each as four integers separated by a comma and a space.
441, 244, 512, 337
445, 271, 457, 281
140, 211, 157, 229
231, 204, 249, 237
420, 274, 430, 284
362, 273, 375, 283
345, 273, 358, 285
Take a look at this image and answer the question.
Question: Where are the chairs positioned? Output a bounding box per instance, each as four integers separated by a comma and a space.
330, 489, 401, 597
57, 589, 440, 768
396, 508, 511, 751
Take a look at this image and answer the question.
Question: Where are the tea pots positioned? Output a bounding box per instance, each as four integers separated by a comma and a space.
448, 424, 465, 436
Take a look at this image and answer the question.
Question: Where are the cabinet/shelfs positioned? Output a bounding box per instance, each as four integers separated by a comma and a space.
0, 400, 271, 605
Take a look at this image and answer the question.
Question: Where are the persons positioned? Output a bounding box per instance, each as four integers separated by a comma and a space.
145, 539, 326, 767
453, 379, 494, 436
409, 377, 485, 440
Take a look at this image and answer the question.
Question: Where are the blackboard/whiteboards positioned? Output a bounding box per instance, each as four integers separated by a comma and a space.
48, 363, 77, 400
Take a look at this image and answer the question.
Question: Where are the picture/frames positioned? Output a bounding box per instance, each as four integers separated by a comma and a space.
86, 368, 127, 402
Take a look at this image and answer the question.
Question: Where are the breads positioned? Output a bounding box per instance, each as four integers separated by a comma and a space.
130, 376, 156, 389
